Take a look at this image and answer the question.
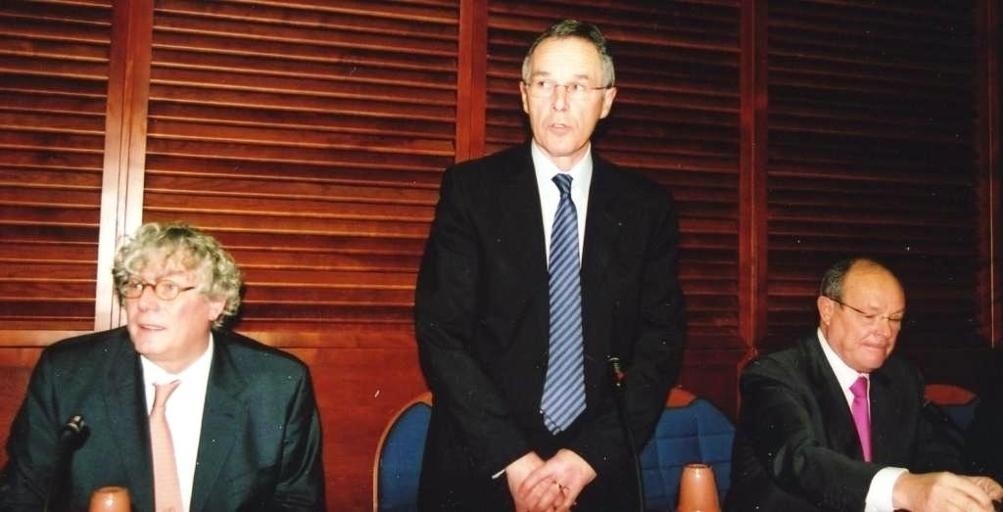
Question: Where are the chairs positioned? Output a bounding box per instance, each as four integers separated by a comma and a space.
919, 385, 981, 480
636, 382, 739, 511
368, 392, 432, 512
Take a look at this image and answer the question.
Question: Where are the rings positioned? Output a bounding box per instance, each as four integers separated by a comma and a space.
554, 481, 564, 489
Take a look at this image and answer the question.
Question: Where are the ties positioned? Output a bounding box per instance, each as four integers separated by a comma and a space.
850, 376, 872, 464
539, 175, 588, 434
141, 380, 186, 512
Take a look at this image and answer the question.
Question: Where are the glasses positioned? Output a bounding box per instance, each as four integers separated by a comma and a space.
828, 294, 902, 328
120, 275, 195, 300
523, 77, 609, 94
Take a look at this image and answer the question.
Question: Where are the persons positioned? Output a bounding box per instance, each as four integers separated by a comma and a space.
416, 16, 686, 512
0, 221, 324, 511
718, 256, 1002, 512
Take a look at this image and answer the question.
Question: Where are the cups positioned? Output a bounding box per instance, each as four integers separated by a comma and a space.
88, 485, 132, 512
676, 463, 722, 512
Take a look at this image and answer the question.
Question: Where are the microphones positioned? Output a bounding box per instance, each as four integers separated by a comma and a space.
605, 356, 644, 512
43, 414, 85, 512
920, 397, 978, 473
